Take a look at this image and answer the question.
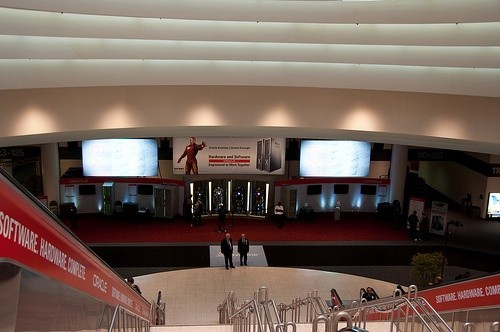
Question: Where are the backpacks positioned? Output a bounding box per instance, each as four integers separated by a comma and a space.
198, 202, 204, 213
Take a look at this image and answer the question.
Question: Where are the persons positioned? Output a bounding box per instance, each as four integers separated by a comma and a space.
127, 276, 134, 286
217, 203, 227, 234
274, 202, 284, 228
184, 194, 194, 224
195, 186, 205, 213
213, 186, 223, 211
254, 187, 264, 214
238, 233, 249, 266
193, 196, 202, 225
419, 212, 430, 237
407, 210, 419, 240
362, 287, 376, 302
233, 185, 244, 214
396, 285, 405, 296
221, 233, 235, 269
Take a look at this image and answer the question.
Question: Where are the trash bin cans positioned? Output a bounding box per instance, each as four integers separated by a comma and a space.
334, 206, 341, 220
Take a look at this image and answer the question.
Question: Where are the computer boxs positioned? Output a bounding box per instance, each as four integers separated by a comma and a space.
255, 137, 282, 172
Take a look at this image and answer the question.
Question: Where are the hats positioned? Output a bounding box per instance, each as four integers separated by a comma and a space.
198, 198, 201, 200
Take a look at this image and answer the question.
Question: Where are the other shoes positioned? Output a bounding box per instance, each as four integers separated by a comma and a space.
244, 264, 247, 265
226, 267, 229, 269
224, 230, 227, 232
218, 231, 221, 233
240, 264, 243, 266
230, 266, 235, 268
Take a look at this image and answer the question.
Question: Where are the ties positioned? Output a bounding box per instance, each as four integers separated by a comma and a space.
369, 293, 370, 295
228, 240, 229, 244
